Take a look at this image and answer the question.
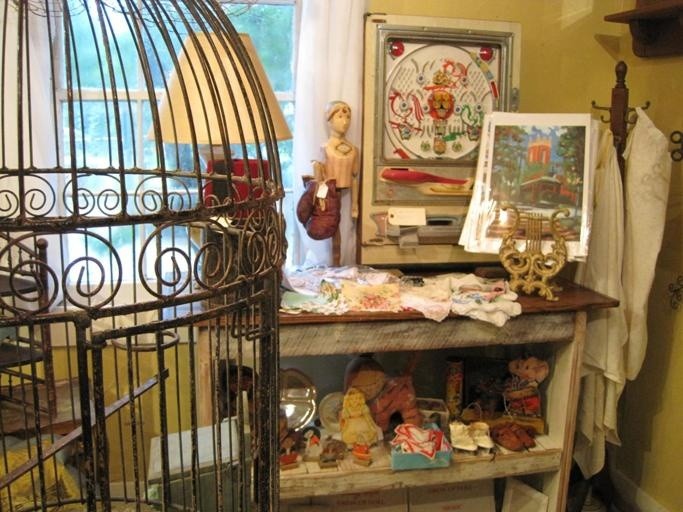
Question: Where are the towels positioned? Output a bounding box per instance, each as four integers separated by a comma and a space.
576, 107, 674, 477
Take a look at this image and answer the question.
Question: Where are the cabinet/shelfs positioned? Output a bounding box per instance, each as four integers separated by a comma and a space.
1, 263, 110, 496
188, 273, 621, 512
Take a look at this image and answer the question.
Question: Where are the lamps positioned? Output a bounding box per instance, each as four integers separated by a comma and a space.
149, 32, 291, 310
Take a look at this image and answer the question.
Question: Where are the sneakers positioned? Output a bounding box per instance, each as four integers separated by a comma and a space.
489, 422, 536, 451
447, 419, 495, 452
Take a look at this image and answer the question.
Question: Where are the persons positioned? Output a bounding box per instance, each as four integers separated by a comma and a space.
337, 388, 385, 450
279, 435, 299, 471
301, 425, 324, 463
310, 97, 362, 219
